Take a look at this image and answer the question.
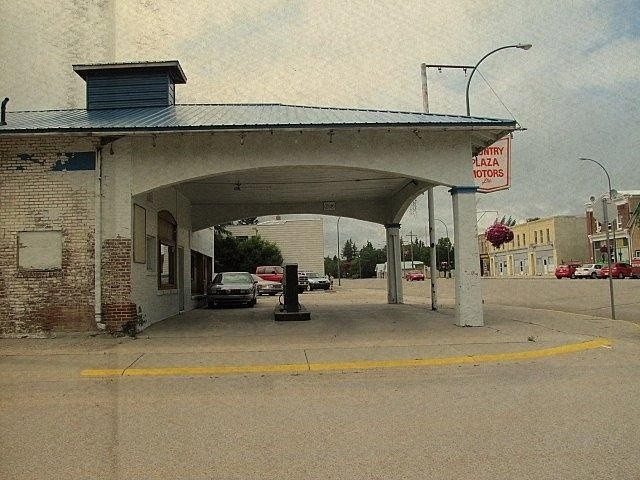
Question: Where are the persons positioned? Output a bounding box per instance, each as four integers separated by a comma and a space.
329, 274, 334, 289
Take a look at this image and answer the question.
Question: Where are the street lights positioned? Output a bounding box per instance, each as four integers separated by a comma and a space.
465, 43, 533, 116
578, 157, 618, 263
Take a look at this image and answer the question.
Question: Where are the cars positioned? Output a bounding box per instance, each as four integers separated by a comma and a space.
206, 265, 331, 308
555, 257, 640, 280
406, 270, 425, 281
